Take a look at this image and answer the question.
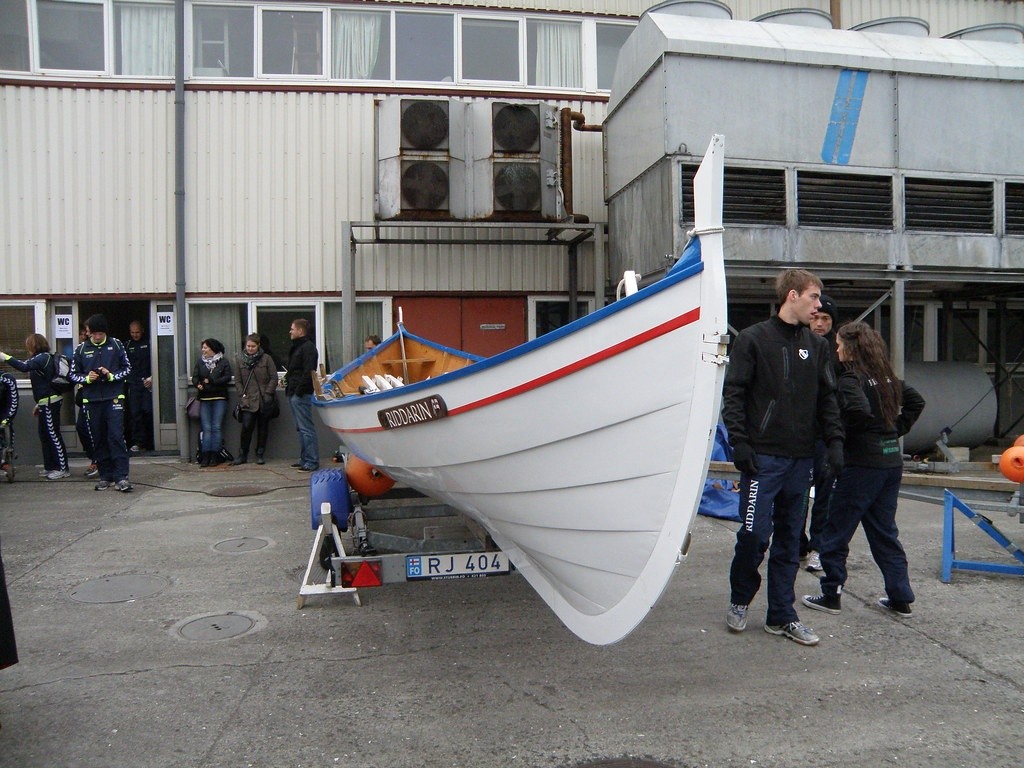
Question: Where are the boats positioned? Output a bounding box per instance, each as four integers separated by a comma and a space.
311, 134, 729, 646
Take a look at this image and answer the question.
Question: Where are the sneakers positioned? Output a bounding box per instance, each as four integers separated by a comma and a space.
802, 594, 842, 615
878, 597, 913, 618
764, 621, 820, 646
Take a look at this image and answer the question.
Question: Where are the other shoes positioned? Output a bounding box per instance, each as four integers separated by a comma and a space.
200, 450, 219, 466
725, 602, 749, 631
95, 480, 116, 490
292, 463, 313, 473
231, 456, 248, 464
131, 445, 140, 451
38, 469, 71, 479
114, 479, 134, 491
804, 550, 823, 571
256, 457, 265, 464
84, 463, 99, 476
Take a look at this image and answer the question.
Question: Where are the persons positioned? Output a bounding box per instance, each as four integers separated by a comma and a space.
722, 269, 845, 646
229, 333, 278, 466
365, 335, 381, 353
192, 339, 230, 468
69, 314, 134, 492
801, 321, 925, 618
279, 318, 322, 473
76, 318, 153, 476
0, 372, 18, 472
798, 293, 839, 571
0, 334, 70, 480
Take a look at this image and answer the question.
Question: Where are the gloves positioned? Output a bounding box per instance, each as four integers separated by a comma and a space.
829, 440, 846, 478
733, 441, 758, 475
0, 352, 6, 362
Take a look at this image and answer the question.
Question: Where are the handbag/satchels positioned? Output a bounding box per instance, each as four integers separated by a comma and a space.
232, 403, 243, 423
261, 397, 280, 420
184, 396, 201, 419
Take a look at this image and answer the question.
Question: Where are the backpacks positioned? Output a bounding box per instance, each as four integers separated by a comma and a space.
34, 351, 71, 387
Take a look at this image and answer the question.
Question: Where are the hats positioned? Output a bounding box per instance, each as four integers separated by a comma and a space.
86, 314, 106, 334
201, 338, 225, 354
816, 295, 838, 328
